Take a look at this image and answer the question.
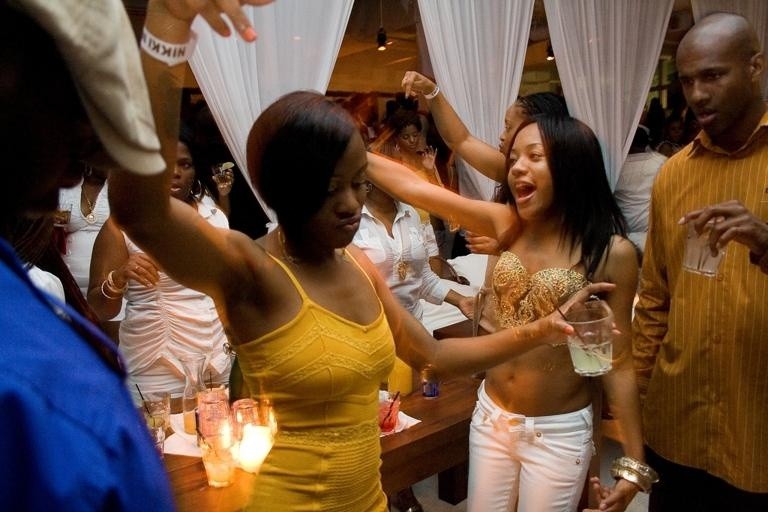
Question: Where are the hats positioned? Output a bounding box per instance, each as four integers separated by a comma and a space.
19, 0, 169, 176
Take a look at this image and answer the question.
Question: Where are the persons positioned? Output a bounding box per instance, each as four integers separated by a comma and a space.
0, 1, 767, 511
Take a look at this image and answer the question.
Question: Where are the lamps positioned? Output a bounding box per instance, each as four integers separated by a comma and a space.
545, 36, 554, 61
376, 1, 387, 51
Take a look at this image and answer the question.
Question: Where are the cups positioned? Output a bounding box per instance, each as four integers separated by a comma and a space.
681, 218, 728, 278
133, 386, 275, 488
378, 393, 401, 433
563, 311, 615, 378
